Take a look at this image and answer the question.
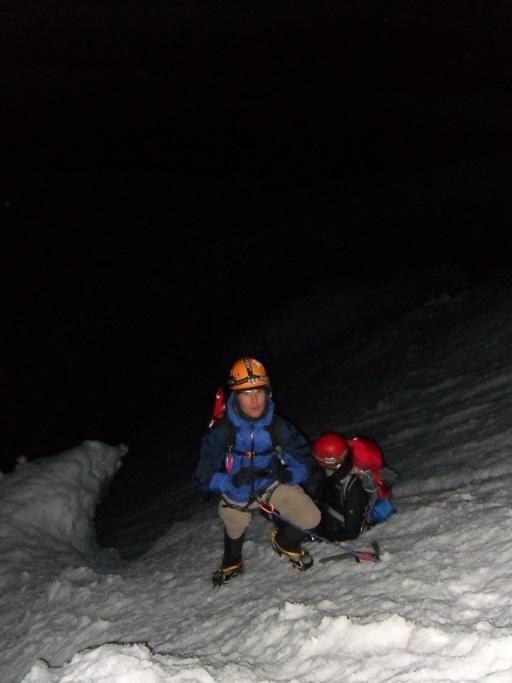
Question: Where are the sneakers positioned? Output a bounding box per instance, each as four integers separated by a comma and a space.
211, 560, 245, 589
270, 527, 314, 572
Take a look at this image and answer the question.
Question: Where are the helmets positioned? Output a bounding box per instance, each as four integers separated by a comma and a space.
311, 430, 347, 468
226, 355, 269, 391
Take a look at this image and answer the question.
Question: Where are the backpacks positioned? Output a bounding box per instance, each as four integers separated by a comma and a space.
345, 434, 401, 532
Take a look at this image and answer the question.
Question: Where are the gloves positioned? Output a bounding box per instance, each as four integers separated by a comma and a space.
275, 465, 293, 483
231, 466, 269, 490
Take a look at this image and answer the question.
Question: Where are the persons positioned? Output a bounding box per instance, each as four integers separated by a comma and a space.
188, 357, 322, 587
259, 432, 398, 543
